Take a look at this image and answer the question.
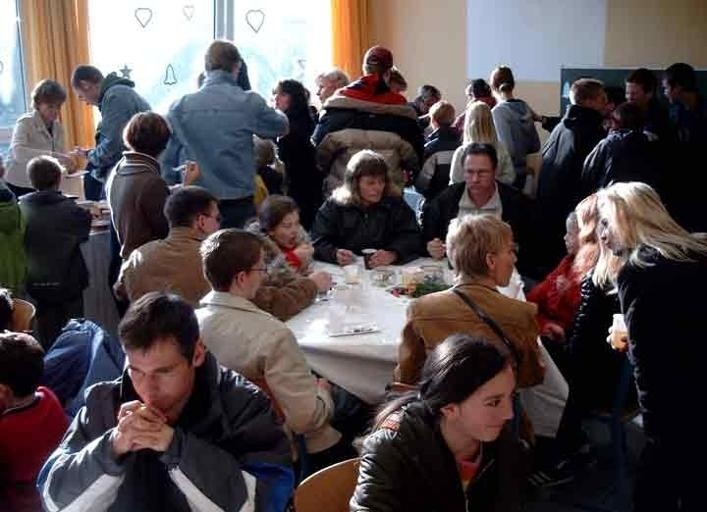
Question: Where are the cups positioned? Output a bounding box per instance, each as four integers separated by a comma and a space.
361, 247, 380, 273
325, 265, 360, 334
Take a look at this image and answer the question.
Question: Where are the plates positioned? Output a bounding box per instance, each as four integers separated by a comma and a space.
66, 199, 109, 228
64, 169, 90, 178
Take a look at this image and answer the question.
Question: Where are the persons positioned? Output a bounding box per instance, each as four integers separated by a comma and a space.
597, 182, 707, 510
346, 334, 545, 508
194, 226, 381, 470
37, 291, 295, 512
0, 43, 707, 423
0, 327, 72, 512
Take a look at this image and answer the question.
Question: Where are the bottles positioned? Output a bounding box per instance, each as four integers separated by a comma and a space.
609, 312, 629, 353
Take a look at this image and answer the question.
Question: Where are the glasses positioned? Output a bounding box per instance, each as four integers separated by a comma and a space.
127, 361, 185, 383
235, 266, 267, 279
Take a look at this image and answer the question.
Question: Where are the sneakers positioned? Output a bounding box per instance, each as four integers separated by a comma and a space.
528, 467, 573, 489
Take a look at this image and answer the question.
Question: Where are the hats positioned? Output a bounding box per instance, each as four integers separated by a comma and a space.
362, 45, 393, 68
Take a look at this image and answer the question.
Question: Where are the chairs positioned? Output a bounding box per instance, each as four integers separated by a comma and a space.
588, 406, 645, 451
42, 316, 104, 410
295, 456, 362, 512
6, 297, 37, 334
